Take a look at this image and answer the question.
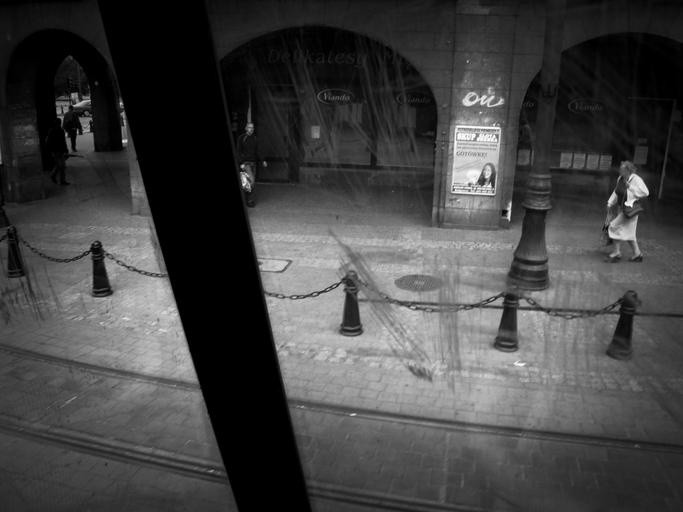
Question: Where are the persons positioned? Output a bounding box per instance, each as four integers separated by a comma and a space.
62, 104, 83, 152
606, 160, 650, 262
233, 122, 268, 208
46, 118, 70, 185
478, 162, 496, 190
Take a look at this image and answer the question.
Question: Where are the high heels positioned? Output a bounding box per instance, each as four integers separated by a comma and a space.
629, 253, 643, 261
607, 253, 622, 260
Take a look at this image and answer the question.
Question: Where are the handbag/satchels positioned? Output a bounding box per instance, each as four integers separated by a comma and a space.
623, 201, 643, 218
603, 215, 616, 229
240, 172, 251, 193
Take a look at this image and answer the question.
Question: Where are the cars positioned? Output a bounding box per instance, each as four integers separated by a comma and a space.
72, 99, 93, 117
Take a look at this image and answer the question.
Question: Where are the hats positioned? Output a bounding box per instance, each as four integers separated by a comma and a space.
621, 161, 637, 172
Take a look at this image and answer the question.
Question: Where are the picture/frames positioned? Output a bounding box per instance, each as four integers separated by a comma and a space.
450, 125, 502, 196
